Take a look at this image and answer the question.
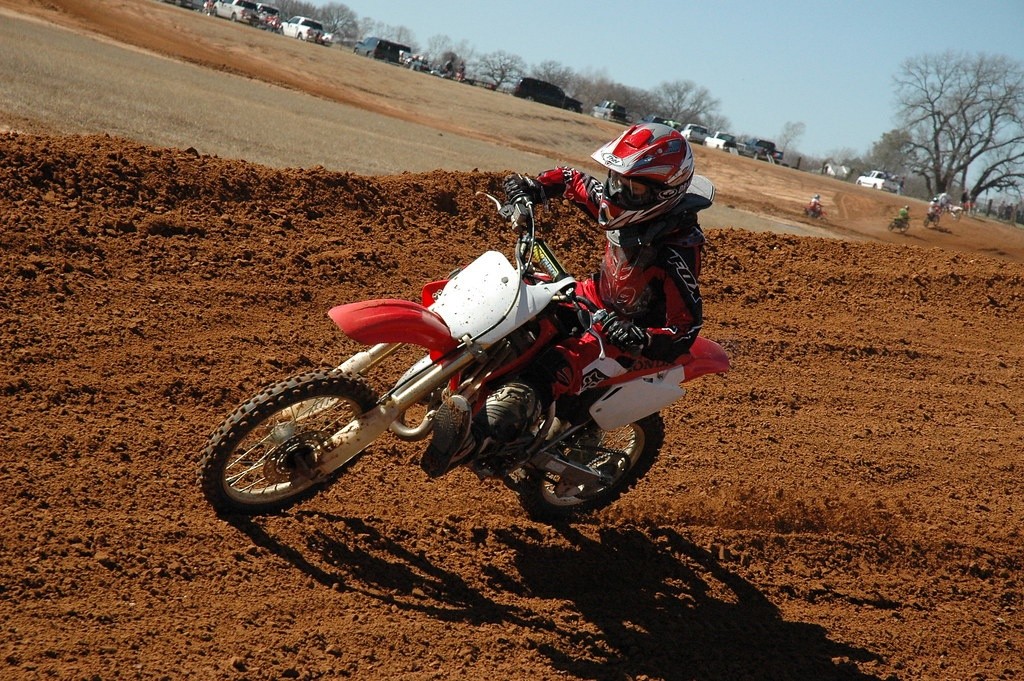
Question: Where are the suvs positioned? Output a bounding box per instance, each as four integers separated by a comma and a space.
512, 77, 583, 114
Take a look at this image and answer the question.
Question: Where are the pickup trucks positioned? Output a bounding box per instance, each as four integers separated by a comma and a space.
279, 15, 333, 47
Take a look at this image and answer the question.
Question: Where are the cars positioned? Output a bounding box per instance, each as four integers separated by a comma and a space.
856, 169, 900, 193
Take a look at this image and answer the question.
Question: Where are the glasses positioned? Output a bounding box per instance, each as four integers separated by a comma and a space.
609, 169, 653, 199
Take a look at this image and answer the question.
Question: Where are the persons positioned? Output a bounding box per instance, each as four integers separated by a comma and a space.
420, 123, 704, 478
898, 205, 911, 228
961, 188, 968, 204
928, 197, 940, 222
809, 194, 823, 212
896, 176, 906, 195
997, 201, 1013, 220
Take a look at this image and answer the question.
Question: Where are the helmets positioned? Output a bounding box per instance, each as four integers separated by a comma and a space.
933, 198, 938, 202
905, 206, 909, 210
591, 122, 695, 230
815, 194, 819, 199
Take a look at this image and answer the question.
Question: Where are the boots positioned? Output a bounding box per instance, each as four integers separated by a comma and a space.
420, 381, 535, 479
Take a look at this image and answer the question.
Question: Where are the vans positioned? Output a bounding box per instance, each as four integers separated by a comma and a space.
353, 37, 411, 64
163, 0, 280, 28
588, 101, 785, 165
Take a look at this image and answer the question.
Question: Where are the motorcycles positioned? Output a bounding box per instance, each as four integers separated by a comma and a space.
923, 205, 943, 227
195, 169, 731, 514
802, 203, 829, 226
888, 215, 912, 233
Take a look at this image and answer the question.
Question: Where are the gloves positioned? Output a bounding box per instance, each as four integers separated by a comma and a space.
503, 173, 541, 205
606, 320, 649, 354
